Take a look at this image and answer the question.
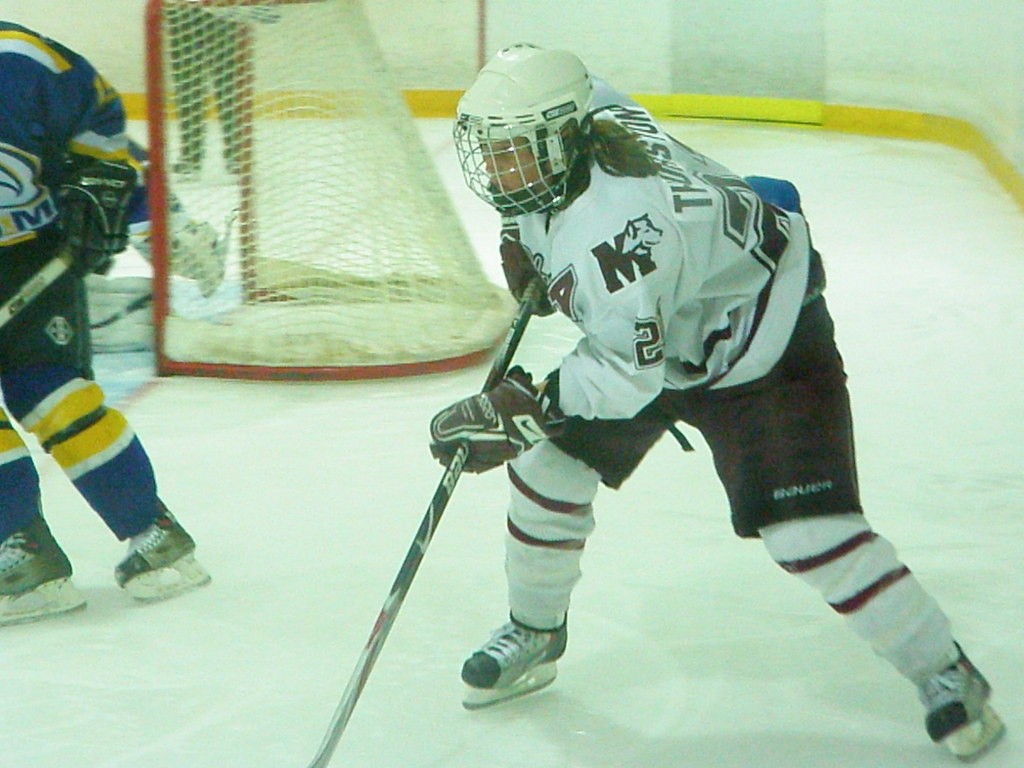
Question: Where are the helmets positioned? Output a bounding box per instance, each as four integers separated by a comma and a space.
452, 44, 593, 218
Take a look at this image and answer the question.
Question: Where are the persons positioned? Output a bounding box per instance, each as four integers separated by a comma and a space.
165, 0, 236, 174
429, 41, 993, 744
1, 20, 221, 598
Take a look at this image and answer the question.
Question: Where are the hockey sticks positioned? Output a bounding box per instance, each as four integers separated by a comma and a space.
306, 274, 544, 767
1, 246, 76, 328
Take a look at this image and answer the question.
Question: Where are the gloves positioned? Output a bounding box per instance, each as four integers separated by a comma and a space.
55, 160, 137, 277
430, 365, 567, 475
502, 227, 557, 316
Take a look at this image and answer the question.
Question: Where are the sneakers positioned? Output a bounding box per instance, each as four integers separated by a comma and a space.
113, 500, 210, 598
918, 642, 1007, 761
462, 610, 565, 707
0, 516, 86, 622
